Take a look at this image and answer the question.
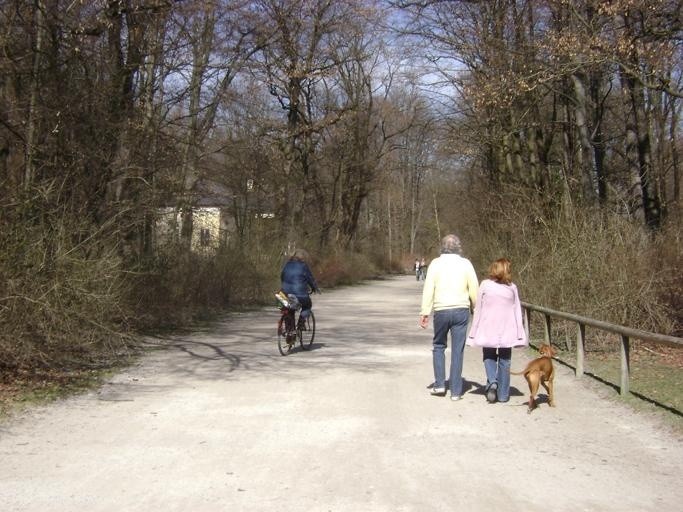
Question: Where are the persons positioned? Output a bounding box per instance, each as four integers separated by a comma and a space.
419, 257, 427, 281
418, 231, 480, 400
413, 257, 420, 281
279, 247, 318, 344
466, 257, 528, 403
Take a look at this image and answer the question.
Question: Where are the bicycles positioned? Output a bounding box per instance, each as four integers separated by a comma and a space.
278, 288, 315, 356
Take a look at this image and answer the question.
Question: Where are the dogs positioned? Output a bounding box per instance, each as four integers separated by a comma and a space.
508, 344, 558, 415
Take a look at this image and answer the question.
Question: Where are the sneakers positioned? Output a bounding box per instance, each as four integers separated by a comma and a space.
430, 387, 460, 400
486, 382, 498, 403
